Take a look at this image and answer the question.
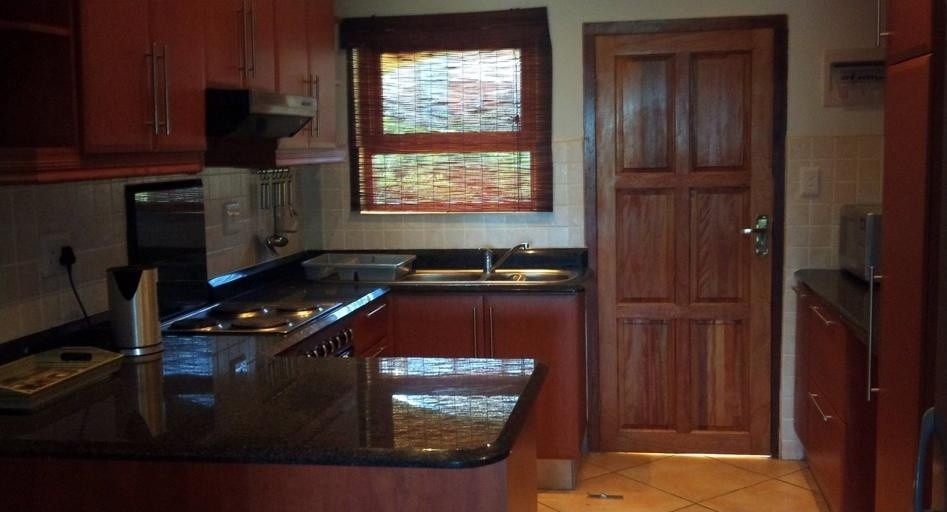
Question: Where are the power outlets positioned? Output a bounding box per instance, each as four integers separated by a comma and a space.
40, 236, 71, 278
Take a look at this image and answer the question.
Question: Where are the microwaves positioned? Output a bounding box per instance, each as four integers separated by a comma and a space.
837, 203, 879, 282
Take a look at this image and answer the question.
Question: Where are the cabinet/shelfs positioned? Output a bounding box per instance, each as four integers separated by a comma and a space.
206, 0, 274, 95
1, 0, 78, 185
790, 278, 875, 512
395, 291, 586, 490
274, 0, 346, 168
78, 0, 206, 180
874, 1, 934, 70
350, 295, 395, 357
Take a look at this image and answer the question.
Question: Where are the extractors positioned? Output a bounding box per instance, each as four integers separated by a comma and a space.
204, 85, 318, 143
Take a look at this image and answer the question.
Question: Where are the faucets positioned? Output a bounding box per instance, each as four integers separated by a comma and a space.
477, 242, 531, 276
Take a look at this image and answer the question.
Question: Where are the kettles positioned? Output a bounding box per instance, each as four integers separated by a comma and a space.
105, 264, 163, 358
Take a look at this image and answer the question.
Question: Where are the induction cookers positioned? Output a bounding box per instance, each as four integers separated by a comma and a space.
160, 299, 341, 335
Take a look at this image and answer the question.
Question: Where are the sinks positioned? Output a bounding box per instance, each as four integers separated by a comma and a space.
396, 268, 578, 286
